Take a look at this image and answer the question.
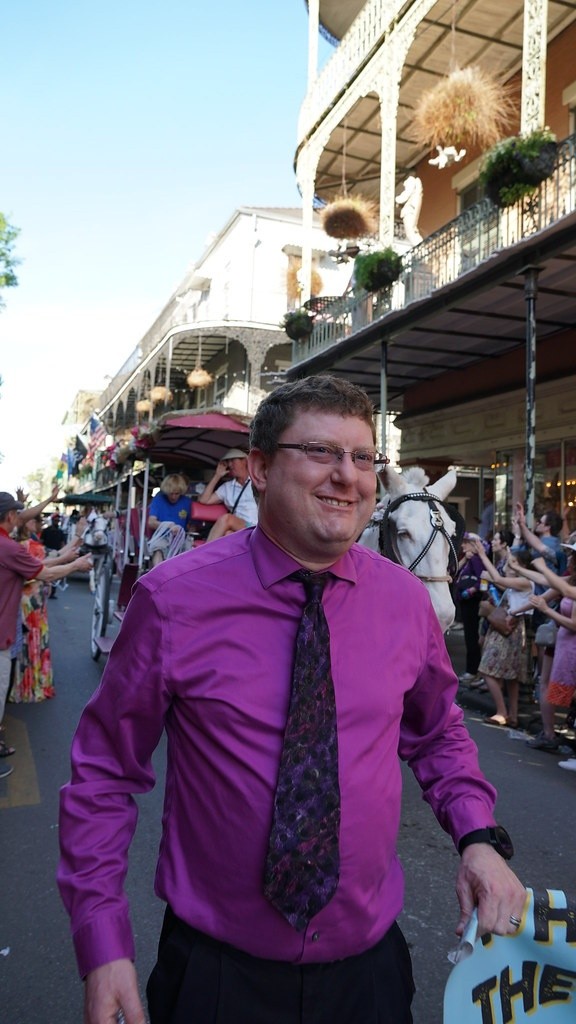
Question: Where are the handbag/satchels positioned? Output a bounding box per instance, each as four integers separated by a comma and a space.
534, 623, 557, 645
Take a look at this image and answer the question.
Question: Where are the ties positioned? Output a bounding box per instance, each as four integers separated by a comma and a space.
263, 568, 341, 932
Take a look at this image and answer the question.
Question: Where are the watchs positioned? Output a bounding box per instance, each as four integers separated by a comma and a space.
458, 826, 514, 861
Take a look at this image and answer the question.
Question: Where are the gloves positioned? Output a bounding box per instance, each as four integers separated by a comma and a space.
462, 589, 472, 599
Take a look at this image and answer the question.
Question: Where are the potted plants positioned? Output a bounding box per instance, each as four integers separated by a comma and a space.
477, 126, 557, 235
347, 245, 407, 293
278, 310, 315, 339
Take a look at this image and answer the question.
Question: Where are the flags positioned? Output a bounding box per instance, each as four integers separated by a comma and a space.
89, 414, 107, 456
71, 436, 88, 477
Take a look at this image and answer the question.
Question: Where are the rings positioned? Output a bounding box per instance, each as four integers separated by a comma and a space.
510, 915, 521, 926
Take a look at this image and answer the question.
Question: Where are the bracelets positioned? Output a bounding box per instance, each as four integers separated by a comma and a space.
515, 536, 522, 539
509, 611, 515, 616
75, 533, 80, 537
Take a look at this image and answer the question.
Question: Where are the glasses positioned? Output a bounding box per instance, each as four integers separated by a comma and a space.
538, 520, 542, 524
54, 519, 60, 522
277, 442, 391, 473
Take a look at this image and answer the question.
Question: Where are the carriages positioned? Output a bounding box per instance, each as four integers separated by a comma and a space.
87, 403, 456, 663
50, 491, 116, 595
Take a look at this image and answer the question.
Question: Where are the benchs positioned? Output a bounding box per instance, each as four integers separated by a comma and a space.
191, 501, 228, 549
131, 508, 152, 557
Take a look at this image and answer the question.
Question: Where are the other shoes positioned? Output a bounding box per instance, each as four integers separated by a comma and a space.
568, 758, 576, 761
457, 672, 477, 682
0, 762, 14, 777
470, 676, 485, 686
479, 684, 488, 690
558, 761, 576, 771
526, 731, 559, 748
554, 727, 575, 736
0, 743, 15, 757
505, 719, 517, 729
48, 595, 58, 599
485, 715, 511, 724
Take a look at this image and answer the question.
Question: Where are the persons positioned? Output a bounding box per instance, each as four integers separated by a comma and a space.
455, 503, 576, 771
147, 473, 192, 568
395, 176, 428, 261
102, 475, 156, 519
57, 374, 526, 1024
0, 483, 101, 779
199, 448, 258, 542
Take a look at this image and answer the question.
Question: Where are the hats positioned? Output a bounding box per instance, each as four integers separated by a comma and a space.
0, 492, 24, 513
560, 543, 576, 551
220, 449, 248, 460
463, 532, 483, 542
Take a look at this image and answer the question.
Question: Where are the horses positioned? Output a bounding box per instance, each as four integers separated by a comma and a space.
77, 513, 110, 594
357, 463, 458, 635
